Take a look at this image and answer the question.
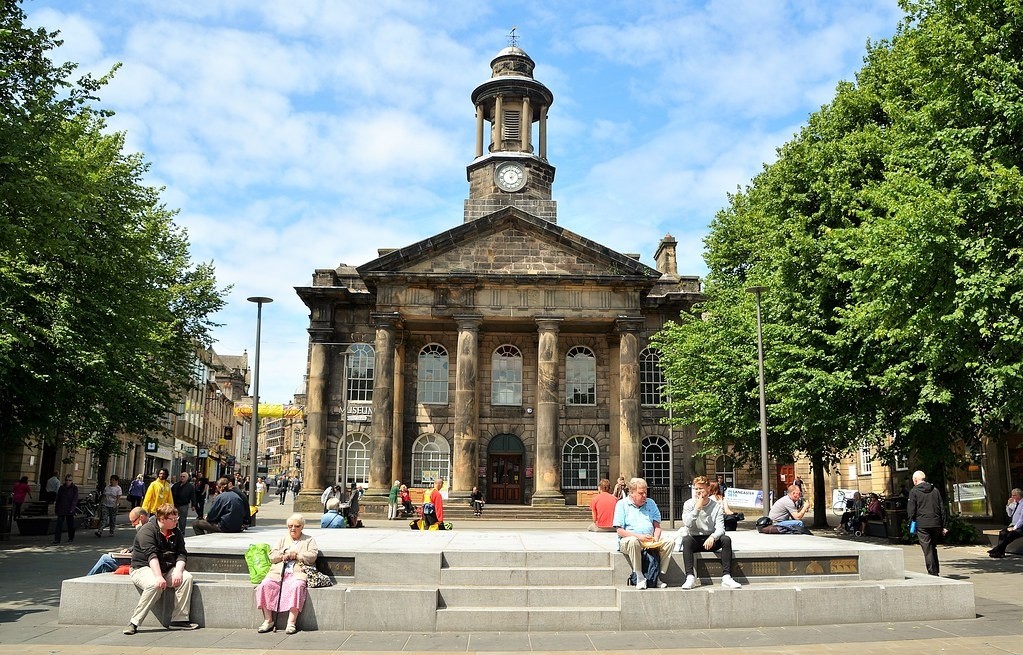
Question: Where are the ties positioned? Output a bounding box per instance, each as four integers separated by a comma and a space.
1011, 503, 1019, 520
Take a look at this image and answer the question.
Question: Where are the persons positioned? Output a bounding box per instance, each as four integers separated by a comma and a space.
858, 493, 882, 535
50, 474, 79, 543
907, 470, 948, 577
470, 487, 486, 518
94, 474, 122, 537
613, 477, 629, 501
86, 507, 150, 575
421, 478, 446, 530
387, 479, 402, 520
987, 489, 1023, 559
255, 512, 319, 633
613, 477, 668, 590
768, 484, 804, 528
716, 475, 726, 498
706, 481, 734, 516
833, 492, 863, 535
12, 476, 33, 521
793, 476, 806, 502
123, 503, 198, 635
587, 479, 619, 532
125, 467, 301, 536
399, 484, 412, 513
321, 482, 366, 528
680, 475, 741, 588
45, 469, 60, 517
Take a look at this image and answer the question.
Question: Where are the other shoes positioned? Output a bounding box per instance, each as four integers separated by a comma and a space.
987, 548, 1006, 559
95, 532, 101, 537
474, 513, 481, 516
833, 526, 865, 536
52, 541, 60, 543
109, 533, 113, 537
68, 539, 72, 542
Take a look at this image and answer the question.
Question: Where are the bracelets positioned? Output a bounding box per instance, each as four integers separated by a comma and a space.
294, 552, 296, 560
280, 555, 283, 560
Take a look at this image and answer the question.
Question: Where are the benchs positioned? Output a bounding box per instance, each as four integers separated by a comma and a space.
982, 529, 1023, 554
867, 510, 902, 538
16, 515, 87, 536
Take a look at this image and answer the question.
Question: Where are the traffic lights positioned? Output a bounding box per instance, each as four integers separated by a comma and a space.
224, 426, 233, 440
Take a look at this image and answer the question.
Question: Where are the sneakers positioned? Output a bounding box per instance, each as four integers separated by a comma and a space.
123, 622, 137, 635
168, 621, 200, 630
286, 622, 297, 634
635, 574, 742, 589
258, 621, 275, 632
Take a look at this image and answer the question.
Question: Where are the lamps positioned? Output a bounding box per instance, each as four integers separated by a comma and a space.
527, 407, 533, 414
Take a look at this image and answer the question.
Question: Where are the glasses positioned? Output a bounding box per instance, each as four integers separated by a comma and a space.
181, 476, 185, 478
288, 524, 302, 529
65, 479, 73, 482
130, 515, 140, 525
164, 516, 180, 523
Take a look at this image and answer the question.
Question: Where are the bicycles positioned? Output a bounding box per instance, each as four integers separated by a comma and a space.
832, 489, 851, 517
73, 491, 110, 529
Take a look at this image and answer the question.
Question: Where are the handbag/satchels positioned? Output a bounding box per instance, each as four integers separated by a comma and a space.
298, 560, 334, 587
909, 520, 917, 534
672, 525, 690, 551
126, 494, 132, 502
245, 542, 272, 583
409, 518, 452, 531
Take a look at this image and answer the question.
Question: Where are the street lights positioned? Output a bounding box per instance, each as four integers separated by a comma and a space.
743, 283, 771, 518
338, 351, 355, 503
247, 296, 273, 527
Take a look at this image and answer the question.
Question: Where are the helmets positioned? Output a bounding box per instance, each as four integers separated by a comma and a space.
756, 516, 773, 533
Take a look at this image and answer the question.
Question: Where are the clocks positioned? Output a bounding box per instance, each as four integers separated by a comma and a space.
493, 161, 527, 192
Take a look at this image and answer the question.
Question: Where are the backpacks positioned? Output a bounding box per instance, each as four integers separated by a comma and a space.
627, 548, 662, 588
